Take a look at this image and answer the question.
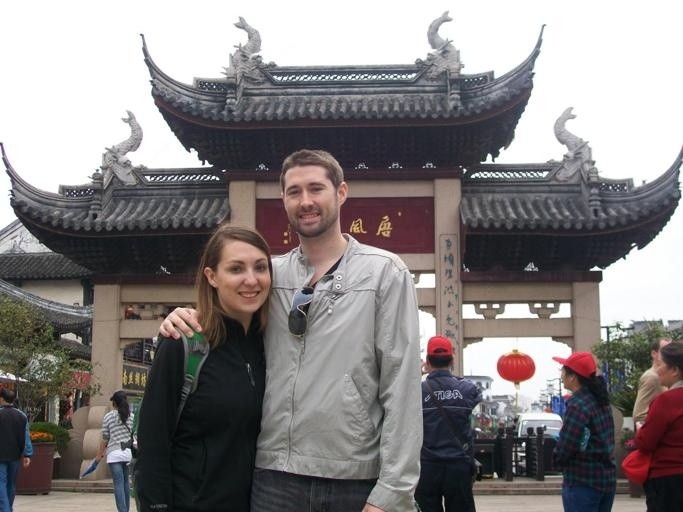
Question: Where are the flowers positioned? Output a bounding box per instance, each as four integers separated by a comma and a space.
621, 432, 635, 450
30, 431, 53, 443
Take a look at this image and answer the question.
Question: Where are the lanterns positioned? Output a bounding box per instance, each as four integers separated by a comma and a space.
497, 349, 536, 407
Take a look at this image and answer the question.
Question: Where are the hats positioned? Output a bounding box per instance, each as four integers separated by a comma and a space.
621, 448, 653, 485
110, 390, 126, 400
427, 337, 454, 357
552, 352, 597, 379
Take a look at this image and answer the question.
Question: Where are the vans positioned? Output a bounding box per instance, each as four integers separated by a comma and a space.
515, 411, 567, 475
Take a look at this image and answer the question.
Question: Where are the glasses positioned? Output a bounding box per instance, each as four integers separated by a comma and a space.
288, 286, 315, 337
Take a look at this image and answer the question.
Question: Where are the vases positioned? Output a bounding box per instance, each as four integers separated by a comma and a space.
629, 481, 642, 497
16, 442, 57, 495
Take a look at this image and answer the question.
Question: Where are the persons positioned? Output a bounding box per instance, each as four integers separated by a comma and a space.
133, 223, 273, 512
635, 341, 683, 512
0, 388, 33, 512
159, 148, 423, 512
95, 389, 135, 512
494, 416, 518, 438
414, 336, 483, 512
552, 351, 617, 512
633, 336, 674, 425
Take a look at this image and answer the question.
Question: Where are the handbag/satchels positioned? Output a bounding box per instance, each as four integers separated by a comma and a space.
472, 457, 483, 487
121, 324, 211, 512
